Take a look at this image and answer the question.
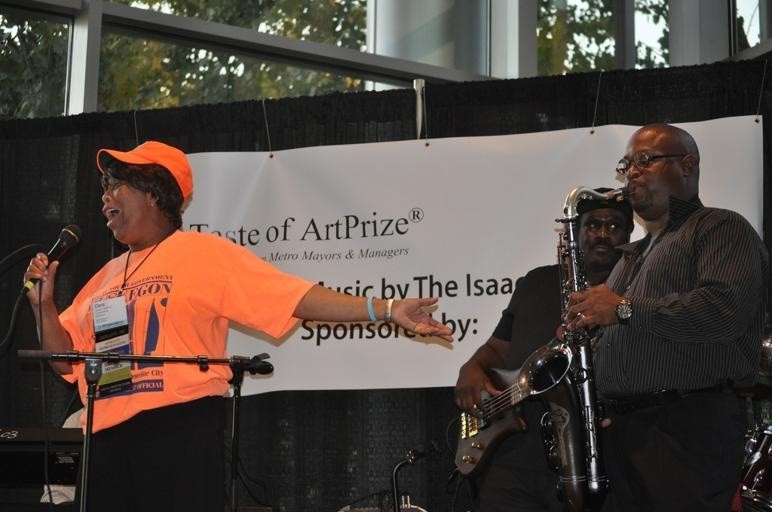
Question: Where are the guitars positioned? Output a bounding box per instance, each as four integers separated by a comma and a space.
454, 368, 528, 477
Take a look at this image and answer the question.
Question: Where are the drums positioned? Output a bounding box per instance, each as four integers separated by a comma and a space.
735, 418, 772, 511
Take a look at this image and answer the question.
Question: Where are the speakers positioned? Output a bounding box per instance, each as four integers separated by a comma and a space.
0, 427, 84, 512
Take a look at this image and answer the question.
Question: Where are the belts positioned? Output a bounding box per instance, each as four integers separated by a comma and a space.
594, 384, 738, 416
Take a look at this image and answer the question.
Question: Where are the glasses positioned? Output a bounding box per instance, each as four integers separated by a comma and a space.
615, 150, 683, 175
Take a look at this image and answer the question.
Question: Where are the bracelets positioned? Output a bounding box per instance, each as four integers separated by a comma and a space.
385, 296, 395, 324
367, 295, 379, 325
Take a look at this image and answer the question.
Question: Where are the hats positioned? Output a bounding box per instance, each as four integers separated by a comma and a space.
96, 141, 193, 203
576, 187, 633, 219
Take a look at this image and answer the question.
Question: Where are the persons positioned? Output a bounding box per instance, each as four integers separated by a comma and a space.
455, 187, 633, 512
23, 140, 453, 512
555, 121, 772, 511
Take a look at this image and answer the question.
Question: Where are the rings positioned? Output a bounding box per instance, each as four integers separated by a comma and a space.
580, 312, 585, 320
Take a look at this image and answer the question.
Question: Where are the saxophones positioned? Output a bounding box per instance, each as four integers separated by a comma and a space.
517, 183, 636, 511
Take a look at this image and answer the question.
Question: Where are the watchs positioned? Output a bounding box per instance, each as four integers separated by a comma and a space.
615, 295, 633, 326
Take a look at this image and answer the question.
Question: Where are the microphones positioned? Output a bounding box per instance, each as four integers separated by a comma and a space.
250, 361, 273, 374
20, 224, 81, 295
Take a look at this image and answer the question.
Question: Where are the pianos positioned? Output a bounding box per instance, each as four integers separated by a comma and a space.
0, 428, 84, 487
